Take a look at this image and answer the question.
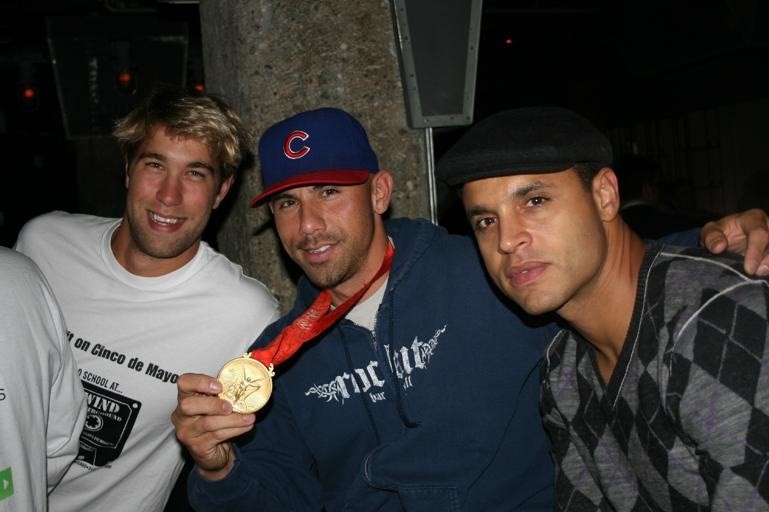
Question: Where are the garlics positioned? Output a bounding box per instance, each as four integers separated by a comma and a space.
213, 355, 274, 416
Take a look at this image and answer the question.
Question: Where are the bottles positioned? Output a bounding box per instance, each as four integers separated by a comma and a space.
248, 107, 379, 208
436, 107, 614, 187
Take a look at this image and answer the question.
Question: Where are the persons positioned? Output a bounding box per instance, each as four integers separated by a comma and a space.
0, 245, 87, 512
172, 106, 769, 509
11, 98, 280, 512
435, 107, 768, 510
